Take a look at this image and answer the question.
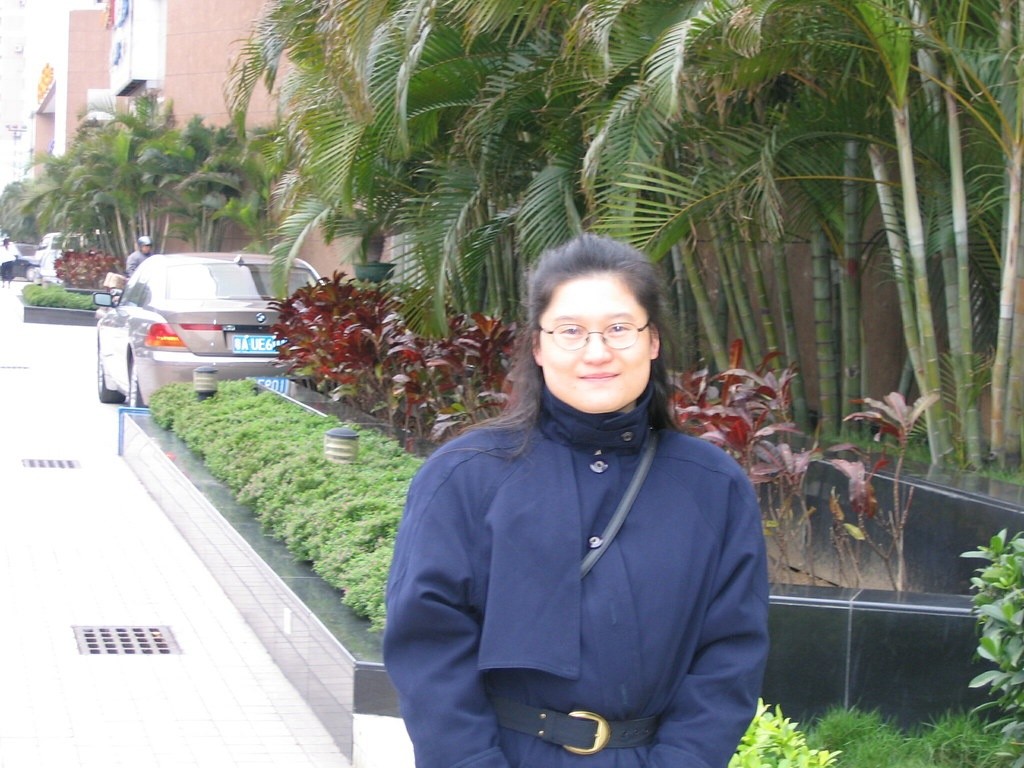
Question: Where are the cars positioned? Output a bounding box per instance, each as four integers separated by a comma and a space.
93, 252, 329, 408
4, 232, 87, 284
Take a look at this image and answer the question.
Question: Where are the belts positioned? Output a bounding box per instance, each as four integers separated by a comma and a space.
487, 691, 661, 755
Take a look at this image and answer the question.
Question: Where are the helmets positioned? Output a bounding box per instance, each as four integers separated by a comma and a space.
138, 236, 152, 254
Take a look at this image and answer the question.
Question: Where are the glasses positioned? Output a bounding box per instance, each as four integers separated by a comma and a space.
536, 317, 651, 350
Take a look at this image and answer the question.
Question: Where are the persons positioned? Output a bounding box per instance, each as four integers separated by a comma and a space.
126, 236, 153, 277
0, 238, 16, 288
384, 235, 771, 768
